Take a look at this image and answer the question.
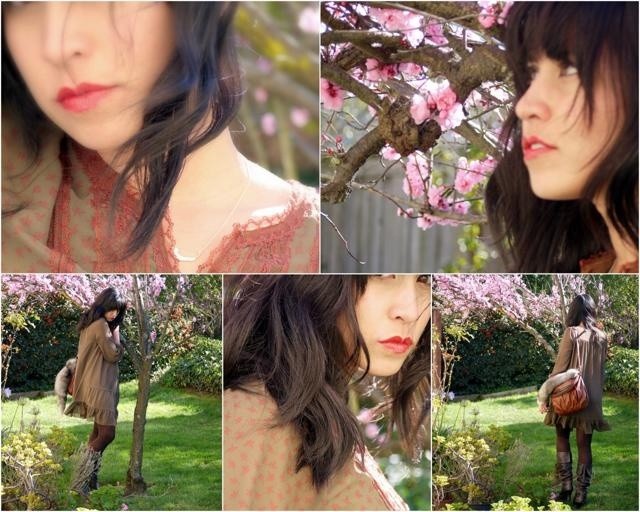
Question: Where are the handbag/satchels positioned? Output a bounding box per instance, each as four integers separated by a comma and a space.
550, 375, 590, 416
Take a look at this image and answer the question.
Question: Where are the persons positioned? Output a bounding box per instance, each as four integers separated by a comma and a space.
539, 294, 611, 506
483, 1, 639, 273
223, 275, 431, 511
1, 1, 320, 274
64, 286, 127, 497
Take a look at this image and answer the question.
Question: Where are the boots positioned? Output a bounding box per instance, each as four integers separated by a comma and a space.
554, 451, 573, 502
573, 464, 592, 509
